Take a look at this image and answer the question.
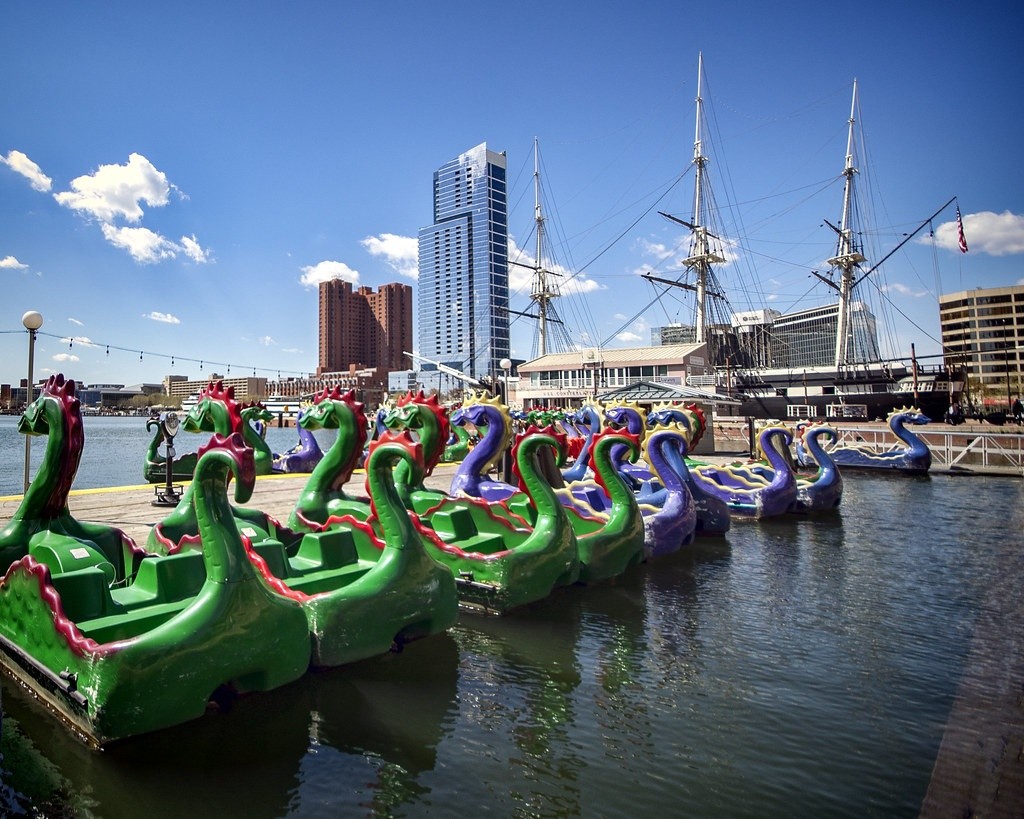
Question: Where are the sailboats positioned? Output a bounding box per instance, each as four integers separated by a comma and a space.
478, 52, 965, 422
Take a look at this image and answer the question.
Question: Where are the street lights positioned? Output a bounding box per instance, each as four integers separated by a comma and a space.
22, 308, 43, 493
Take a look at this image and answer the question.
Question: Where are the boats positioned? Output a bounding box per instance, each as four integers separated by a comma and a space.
147, 382, 459, 670
385, 390, 843, 595
273, 401, 582, 473
144, 401, 273, 482
795, 405, 932, 476
0, 374, 310, 750
